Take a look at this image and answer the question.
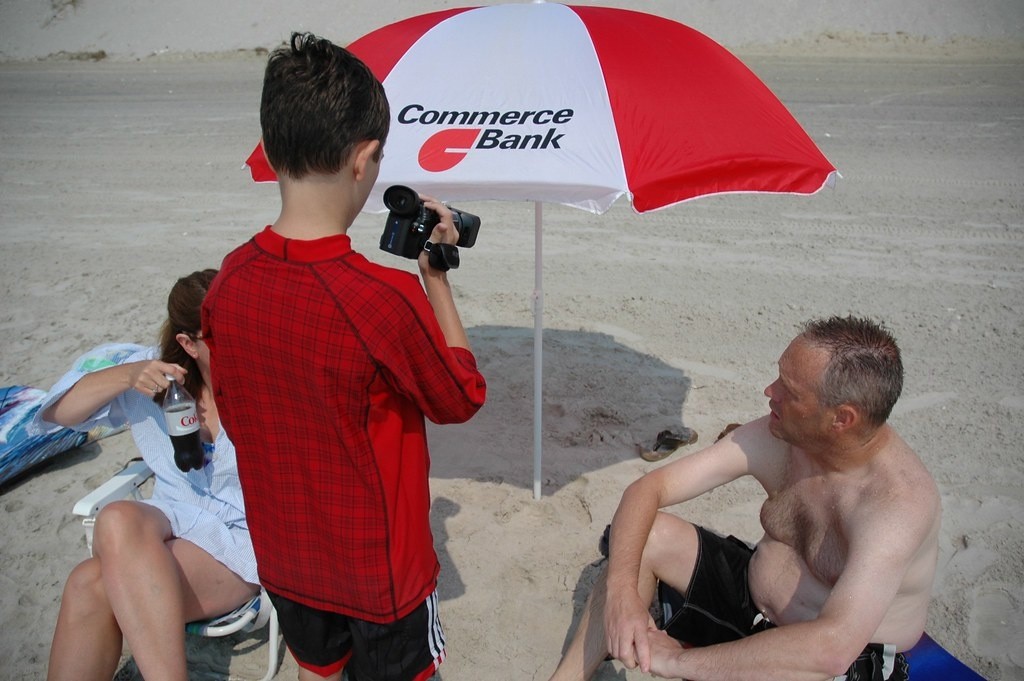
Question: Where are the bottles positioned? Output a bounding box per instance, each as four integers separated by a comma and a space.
163, 372, 204, 472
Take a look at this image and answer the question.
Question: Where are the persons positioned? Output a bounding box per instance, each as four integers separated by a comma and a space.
550, 316, 939, 679
33, 269, 261, 681
200, 32, 488, 681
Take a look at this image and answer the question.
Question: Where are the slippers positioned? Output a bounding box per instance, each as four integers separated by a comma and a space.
640, 427, 698, 462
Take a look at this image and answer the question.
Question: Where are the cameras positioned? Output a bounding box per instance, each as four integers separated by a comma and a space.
380, 185, 482, 261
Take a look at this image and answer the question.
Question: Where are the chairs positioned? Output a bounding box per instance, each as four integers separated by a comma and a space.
70, 457, 279, 681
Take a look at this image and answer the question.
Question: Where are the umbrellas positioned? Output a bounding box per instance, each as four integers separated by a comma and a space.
242, 0, 842, 501
